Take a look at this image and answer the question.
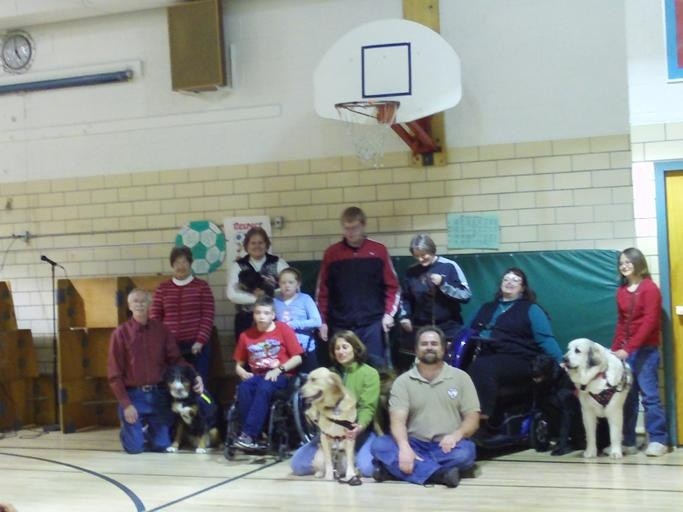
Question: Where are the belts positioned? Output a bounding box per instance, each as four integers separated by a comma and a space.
129, 384, 164, 393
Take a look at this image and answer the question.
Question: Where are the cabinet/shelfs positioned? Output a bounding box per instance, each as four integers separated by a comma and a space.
55, 274, 175, 435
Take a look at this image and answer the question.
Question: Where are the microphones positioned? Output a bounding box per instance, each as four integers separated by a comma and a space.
40, 255, 58, 266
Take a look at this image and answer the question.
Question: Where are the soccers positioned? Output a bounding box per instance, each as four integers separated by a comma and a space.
175, 220, 226, 275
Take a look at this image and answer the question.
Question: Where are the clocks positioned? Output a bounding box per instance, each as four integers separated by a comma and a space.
0, 29, 37, 76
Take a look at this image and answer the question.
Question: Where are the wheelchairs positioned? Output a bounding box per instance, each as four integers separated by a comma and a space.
444, 332, 551, 452
223, 325, 319, 459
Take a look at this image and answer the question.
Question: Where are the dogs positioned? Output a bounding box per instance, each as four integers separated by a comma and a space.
561, 338, 633, 458
297, 366, 363, 485
160, 363, 220, 455
529, 355, 611, 456
372, 369, 398, 436
239, 269, 276, 311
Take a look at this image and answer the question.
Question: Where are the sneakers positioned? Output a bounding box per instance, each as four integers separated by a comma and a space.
437, 467, 460, 486
372, 465, 395, 481
644, 443, 667, 456
233, 433, 255, 449
604, 443, 635, 455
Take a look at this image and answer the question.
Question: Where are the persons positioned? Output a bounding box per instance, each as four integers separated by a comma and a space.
461, 267, 565, 371
105, 287, 205, 455
314, 206, 402, 374
370, 324, 482, 488
149, 245, 215, 380
288, 328, 381, 477
226, 226, 324, 449
397, 232, 472, 367
601, 246, 670, 458
